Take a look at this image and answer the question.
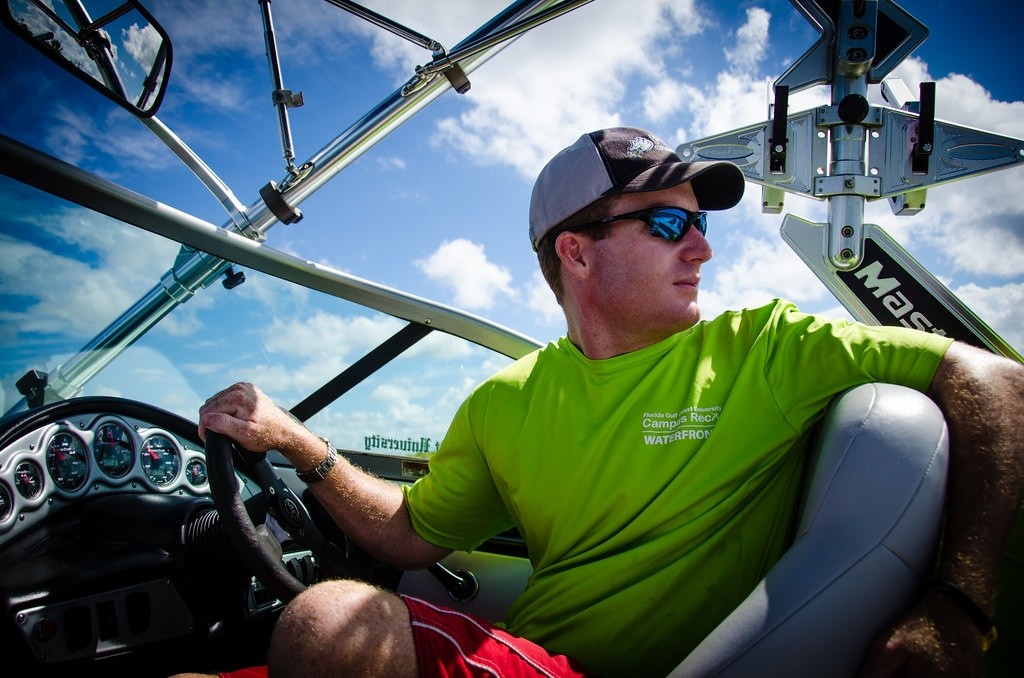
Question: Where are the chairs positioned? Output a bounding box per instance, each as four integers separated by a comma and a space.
666, 382, 951, 678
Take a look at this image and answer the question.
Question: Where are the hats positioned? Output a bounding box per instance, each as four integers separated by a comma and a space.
529, 126, 745, 252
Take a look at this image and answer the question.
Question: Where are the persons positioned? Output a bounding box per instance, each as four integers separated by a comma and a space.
198, 126, 1024, 678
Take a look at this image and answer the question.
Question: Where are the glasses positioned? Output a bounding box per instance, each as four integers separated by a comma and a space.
593, 206, 707, 241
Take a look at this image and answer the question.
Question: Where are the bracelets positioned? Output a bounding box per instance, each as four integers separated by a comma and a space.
296, 436, 337, 484
